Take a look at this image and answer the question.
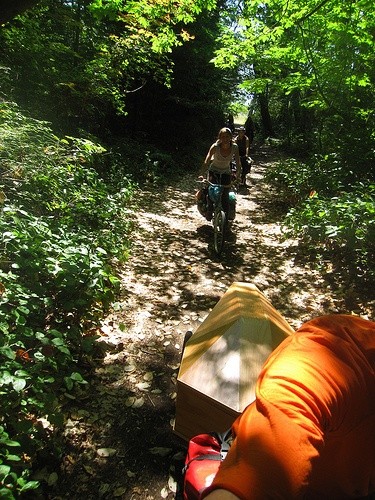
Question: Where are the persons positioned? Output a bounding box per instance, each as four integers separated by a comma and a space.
197, 127, 242, 222
197, 312, 375, 500
234, 126, 249, 158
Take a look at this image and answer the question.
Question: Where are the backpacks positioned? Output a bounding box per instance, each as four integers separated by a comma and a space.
195, 184, 214, 222
185, 427, 236, 500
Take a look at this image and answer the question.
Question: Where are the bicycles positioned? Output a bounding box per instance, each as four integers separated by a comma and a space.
197, 177, 235, 254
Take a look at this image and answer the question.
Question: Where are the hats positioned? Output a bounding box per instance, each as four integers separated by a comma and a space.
237, 126, 246, 131
